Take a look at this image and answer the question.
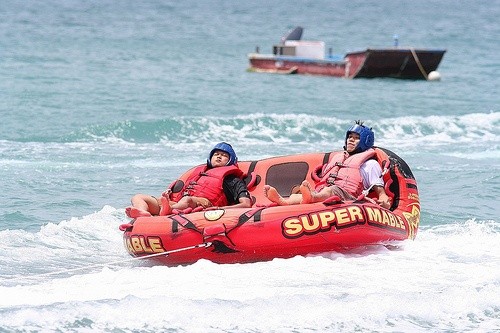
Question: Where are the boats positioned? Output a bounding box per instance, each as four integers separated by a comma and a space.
118, 147, 421, 264
246, 27, 447, 80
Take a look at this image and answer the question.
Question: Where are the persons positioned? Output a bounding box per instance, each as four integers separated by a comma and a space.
125, 141, 251, 217
263, 122, 391, 209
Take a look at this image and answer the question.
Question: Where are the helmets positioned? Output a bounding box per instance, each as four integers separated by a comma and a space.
345, 122, 375, 154
207, 142, 236, 166
